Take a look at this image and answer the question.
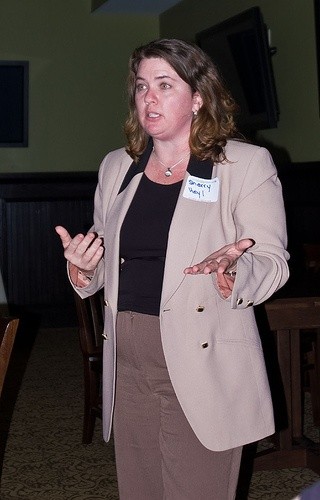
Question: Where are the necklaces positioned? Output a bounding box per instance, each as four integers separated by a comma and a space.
153, 146, 189, 177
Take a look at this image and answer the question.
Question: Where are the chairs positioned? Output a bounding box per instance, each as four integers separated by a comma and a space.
73, 284, 103, 445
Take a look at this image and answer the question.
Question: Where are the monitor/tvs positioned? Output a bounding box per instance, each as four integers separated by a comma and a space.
199, 5, 281, 135
0, 62, 30, 148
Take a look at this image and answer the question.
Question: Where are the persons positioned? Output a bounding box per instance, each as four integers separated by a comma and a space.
56, 40, 289, 500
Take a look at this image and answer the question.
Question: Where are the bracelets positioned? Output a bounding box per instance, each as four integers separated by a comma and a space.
224, 270, 237, 276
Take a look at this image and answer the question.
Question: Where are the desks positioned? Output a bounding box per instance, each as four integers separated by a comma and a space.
253, 297, 320, 475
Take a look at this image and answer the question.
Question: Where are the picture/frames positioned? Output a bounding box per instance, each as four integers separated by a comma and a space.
0, 60, 29, 148
